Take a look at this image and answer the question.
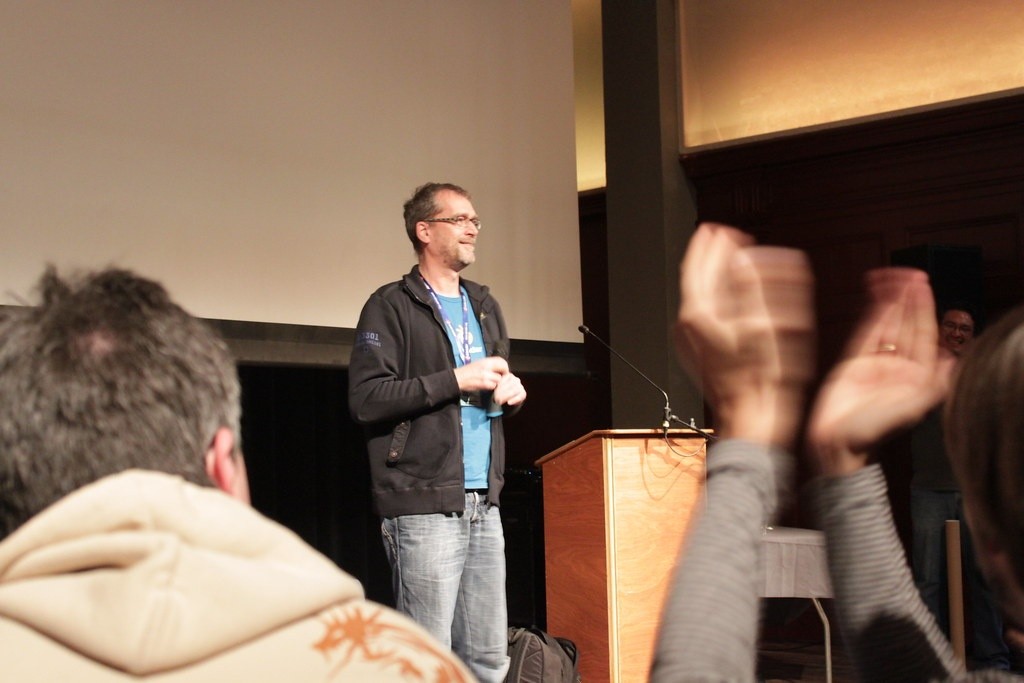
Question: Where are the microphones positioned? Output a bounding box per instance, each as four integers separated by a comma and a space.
578, 324, 670, 432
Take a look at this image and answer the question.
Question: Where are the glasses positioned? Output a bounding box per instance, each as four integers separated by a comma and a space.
424, 216, 482, 230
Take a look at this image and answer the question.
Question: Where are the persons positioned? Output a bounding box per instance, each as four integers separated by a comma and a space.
910, 306, 1023, 675
348, 182, 526, 683
645, 223, 971, 683
0, 271, 478, 683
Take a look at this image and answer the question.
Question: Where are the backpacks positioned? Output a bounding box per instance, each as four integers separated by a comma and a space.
506, 625, 580, 683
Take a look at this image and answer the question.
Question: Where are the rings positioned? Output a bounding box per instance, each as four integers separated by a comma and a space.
878, 343, 895, 351
